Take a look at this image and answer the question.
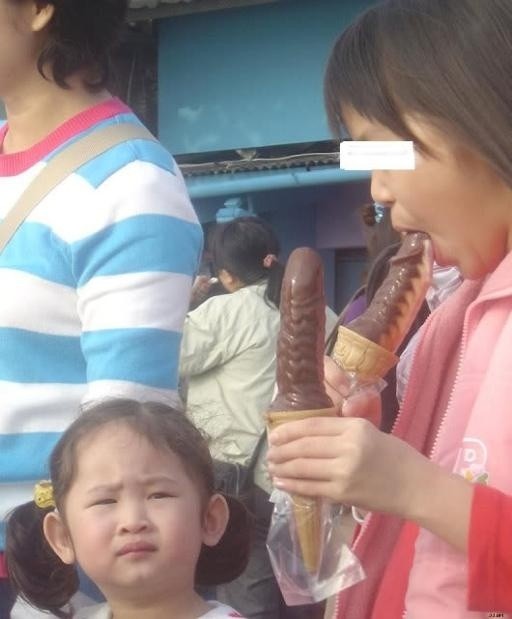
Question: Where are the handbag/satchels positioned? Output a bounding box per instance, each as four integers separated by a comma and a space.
212, 461, 256, 515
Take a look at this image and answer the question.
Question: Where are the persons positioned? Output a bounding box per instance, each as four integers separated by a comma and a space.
0, 0, 209, 618
267, 1, 512, 617
172, 215, 348, 508
4, 394, 251, 618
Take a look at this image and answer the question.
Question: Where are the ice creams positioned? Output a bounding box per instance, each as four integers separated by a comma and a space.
268, 247, 336, 576
326, 231, 435, 392
